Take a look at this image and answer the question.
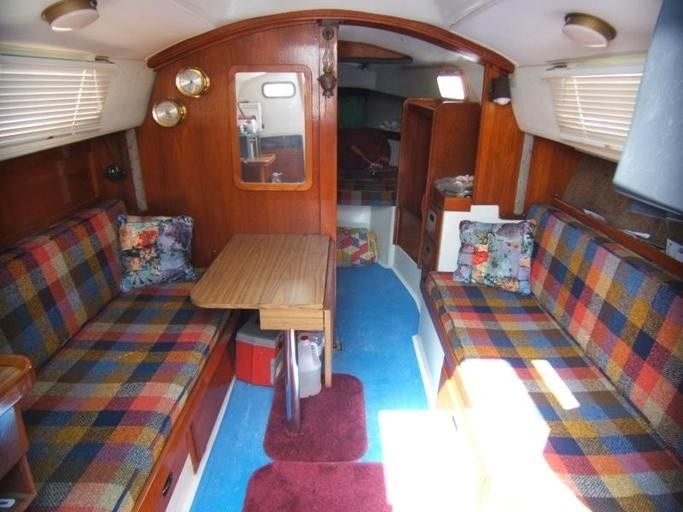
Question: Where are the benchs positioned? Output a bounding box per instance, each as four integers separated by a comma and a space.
412, 192, 682, 511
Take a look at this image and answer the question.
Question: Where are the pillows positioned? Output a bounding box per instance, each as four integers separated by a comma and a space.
452, 220, 536, 295
117, 214, 199, 292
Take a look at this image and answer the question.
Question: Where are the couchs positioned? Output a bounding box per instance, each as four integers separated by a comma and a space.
0, 200, 244, 512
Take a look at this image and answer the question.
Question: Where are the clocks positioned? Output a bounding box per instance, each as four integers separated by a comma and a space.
151, 65, 210, 129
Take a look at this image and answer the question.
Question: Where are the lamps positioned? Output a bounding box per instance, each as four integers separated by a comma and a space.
436, 68, 471, 102
41, 0, 101, 33
561, 13, 617, 48
491, 74, 512, 106
317, 28, 339, 99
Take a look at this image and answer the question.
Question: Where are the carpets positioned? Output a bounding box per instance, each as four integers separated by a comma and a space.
243, 373, 391, 512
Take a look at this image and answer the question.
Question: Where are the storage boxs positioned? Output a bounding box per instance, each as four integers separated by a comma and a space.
234, 310, 284, 388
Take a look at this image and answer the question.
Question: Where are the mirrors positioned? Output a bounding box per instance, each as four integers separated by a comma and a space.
229, 64, 313, 191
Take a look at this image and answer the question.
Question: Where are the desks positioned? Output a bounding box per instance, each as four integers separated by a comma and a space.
190, 232, 337, 433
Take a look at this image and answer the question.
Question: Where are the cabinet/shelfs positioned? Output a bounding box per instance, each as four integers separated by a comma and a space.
139, 417, 189, 512
392, 98, 480, 268
